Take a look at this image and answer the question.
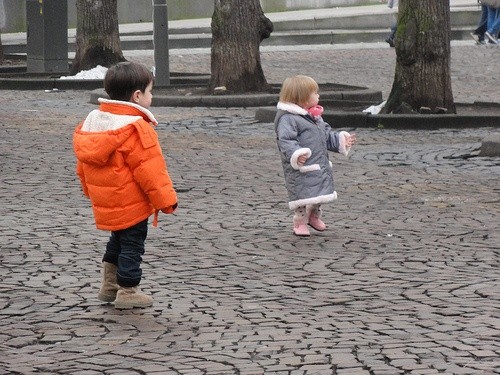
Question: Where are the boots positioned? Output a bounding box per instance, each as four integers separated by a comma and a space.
97, 262, 120, 302
116, 284, 153, 309
307, 207, 325, 231
293, 215, 310, 236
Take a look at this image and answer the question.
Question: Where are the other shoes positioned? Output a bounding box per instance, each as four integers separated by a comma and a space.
385, 37, 395, 47
486, 32, 497, 44
470, 32, 481, 45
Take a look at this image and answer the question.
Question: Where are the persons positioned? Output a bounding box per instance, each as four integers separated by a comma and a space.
274, 75, 355, 238
470, 0, 487, 45
384, 0, 399, 48
485, 0, 500, 44
73, 62, 178, 310
